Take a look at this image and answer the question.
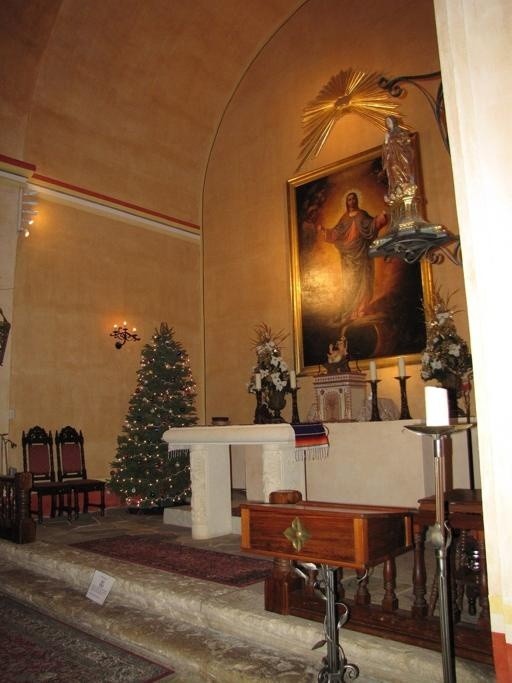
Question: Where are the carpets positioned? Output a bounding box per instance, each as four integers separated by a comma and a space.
69, 532, 274, 588
1, 592, 178, 683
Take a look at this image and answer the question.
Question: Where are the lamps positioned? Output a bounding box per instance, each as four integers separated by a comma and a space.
17, 185, 40, 238
111, 318, 141, 349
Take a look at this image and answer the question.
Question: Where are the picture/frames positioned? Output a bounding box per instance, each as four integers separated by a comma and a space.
285, 130, 434, 376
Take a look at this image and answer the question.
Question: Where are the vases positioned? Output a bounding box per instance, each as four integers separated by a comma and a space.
268, 391, 287, 422
440, 373, 466, 416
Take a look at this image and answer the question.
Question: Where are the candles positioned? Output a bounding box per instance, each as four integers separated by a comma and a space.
424, 385, 449, 426
255, 372, 261, 388
369, 360, 377, 380
290, 369, 297, 388
398, 358, 405, 377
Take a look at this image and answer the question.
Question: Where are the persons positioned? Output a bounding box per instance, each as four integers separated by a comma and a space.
380, 113, 434, 236
316, 192, 390, 325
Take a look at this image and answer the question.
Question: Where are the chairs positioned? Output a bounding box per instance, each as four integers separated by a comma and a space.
22, 424, 106, 524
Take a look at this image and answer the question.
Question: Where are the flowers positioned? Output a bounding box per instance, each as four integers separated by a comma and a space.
247, 321, 293, 394
414, 274, 472, 380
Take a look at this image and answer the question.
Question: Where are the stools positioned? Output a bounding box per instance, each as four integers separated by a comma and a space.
417, 487, 483, 614
